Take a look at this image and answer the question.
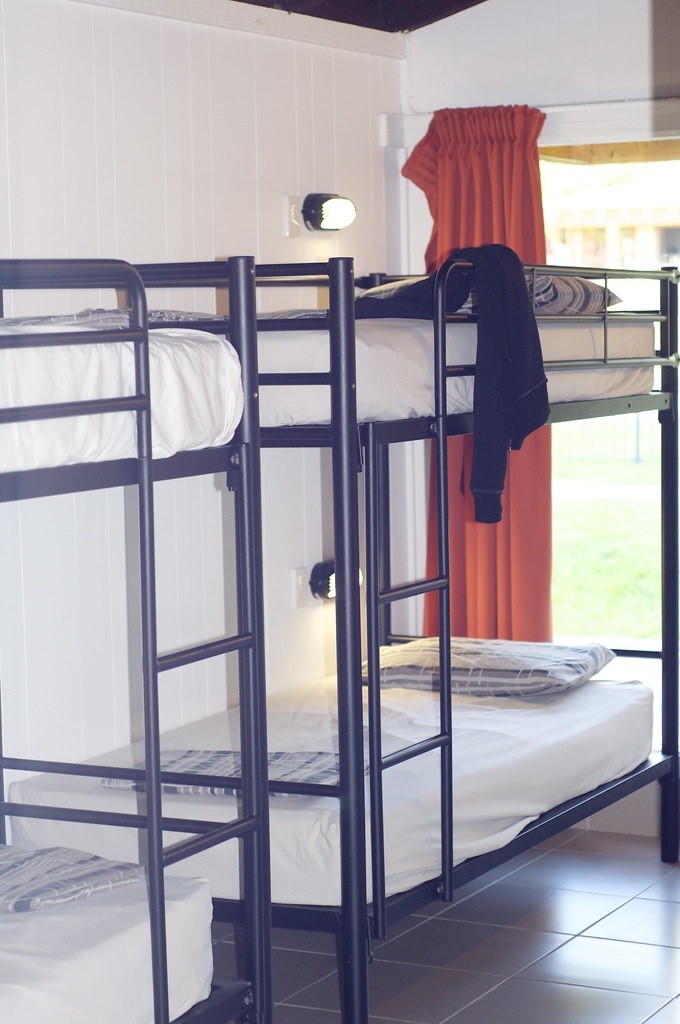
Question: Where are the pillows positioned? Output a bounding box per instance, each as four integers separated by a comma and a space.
358, 275, 623, 315
362, 637, 617, 696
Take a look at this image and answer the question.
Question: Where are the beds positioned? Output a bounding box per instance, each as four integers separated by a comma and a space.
0, 256, 679, 1024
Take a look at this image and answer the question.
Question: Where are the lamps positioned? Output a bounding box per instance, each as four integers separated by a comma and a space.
302, 193, 356, 231
